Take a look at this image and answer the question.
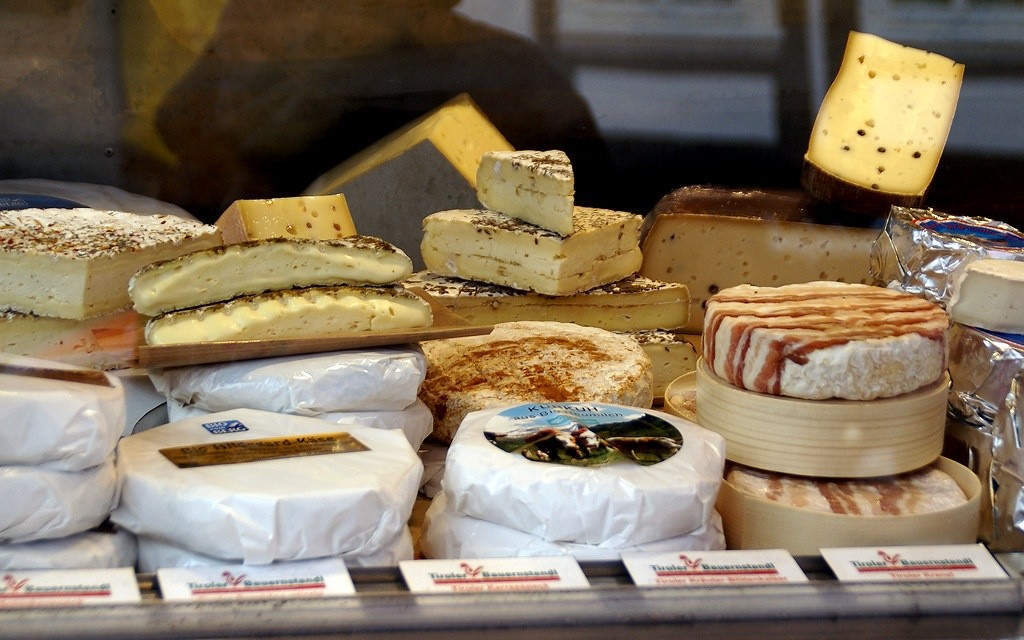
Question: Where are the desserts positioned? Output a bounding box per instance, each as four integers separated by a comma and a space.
0, 30, 1024, 518
